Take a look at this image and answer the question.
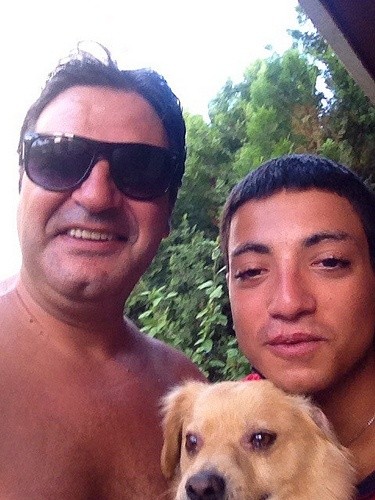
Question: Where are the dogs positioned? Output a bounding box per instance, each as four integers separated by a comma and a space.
155, 375, 361, 500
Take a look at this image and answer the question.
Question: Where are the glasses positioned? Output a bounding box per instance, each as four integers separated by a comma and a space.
22, 134, 178, 200
